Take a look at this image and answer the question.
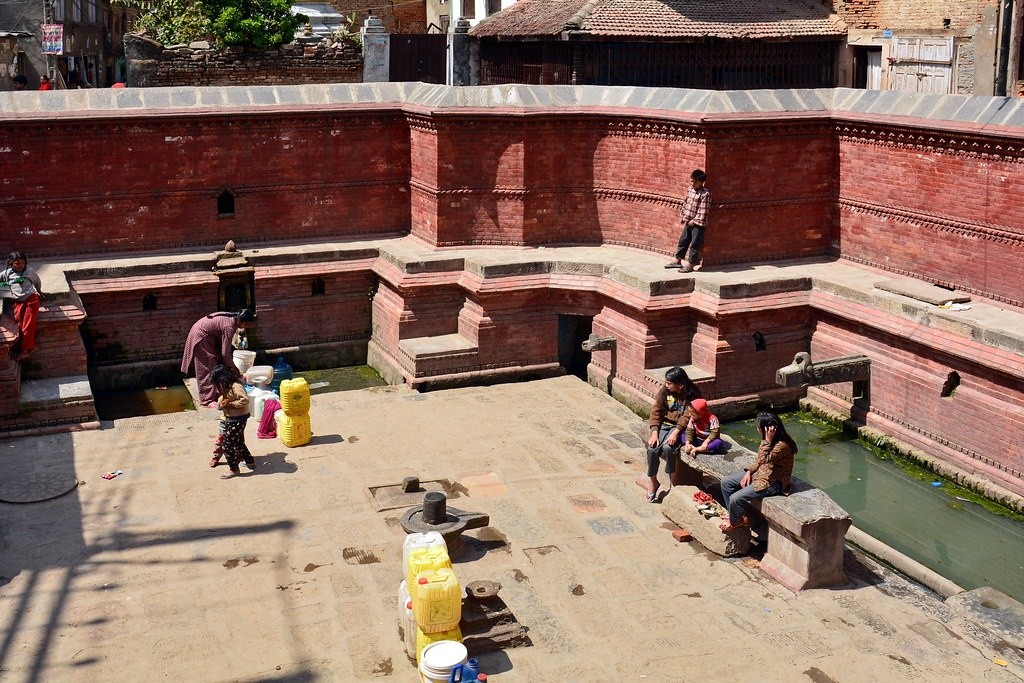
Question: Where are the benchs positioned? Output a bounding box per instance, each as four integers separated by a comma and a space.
629, 418, 853, 593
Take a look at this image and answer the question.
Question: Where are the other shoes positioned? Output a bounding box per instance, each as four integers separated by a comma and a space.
719, 514, 749, 532
15, 347, 38, 361
239, 461, 255, 469
209, 456, 220, 467
220, 470, 240, 479
203, 400, 218, 408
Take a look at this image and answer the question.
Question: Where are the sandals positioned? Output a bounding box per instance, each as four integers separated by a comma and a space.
698, 494, 716, 504
693, 491, 705, 501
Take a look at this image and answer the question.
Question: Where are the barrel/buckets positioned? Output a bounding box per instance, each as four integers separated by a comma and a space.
231, 340, 311, 448
398, 531, 468, 683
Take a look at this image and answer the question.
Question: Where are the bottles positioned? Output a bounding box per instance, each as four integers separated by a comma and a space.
465, 658, 488, 683
269, 357, 293, 396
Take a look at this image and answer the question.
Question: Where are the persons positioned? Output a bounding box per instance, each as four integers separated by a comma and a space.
681, 399, 723, 459
13, 74, 28, 91
645, 368, 702, 503
0, 252, 45, 361
208, 364, 256, 479
38, 75, 53, 90
181, 308, 254, 410
719, 411, 798, 531
664, 169, 712, 273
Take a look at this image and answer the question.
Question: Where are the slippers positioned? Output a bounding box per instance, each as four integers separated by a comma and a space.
664, 261, 683, 267
695, 501, 727, 516
678, 265, 694, 273
645, 483, 660, 502
